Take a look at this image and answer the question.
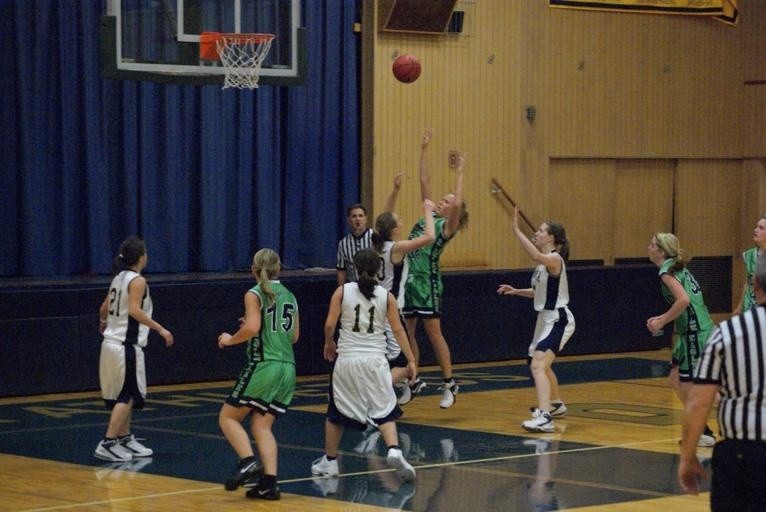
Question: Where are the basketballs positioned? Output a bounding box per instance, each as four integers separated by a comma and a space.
391, 54, 423, 85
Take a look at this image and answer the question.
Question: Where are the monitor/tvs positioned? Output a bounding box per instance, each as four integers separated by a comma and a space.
379, 0, 460, 36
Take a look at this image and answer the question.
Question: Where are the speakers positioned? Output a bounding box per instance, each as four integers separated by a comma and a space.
448, 9, 463, 32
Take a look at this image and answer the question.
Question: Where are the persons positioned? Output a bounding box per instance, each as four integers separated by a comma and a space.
94, 234, 174, 462
370, 171, 436, 384
728, 216, 766, 316
311, 248, 416, 482
400, 125, 468, 409
646, 232, 726, 447
218, 247, 300, 501
336, 204, 378, 286
679, 250, 766, 512
496, 204, 576, 433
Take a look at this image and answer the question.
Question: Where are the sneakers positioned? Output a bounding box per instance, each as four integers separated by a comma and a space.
441, 438, 460, 465
386, 448, 417, 483
399, 376, 426, 405
94, 462, 130, 482
310, 456, 339, 476
354, 431, 381, 454
521, 411, 556, 434
128, 458, 154, 474
680, 435, 716, 448
310, 476, 339, 497
399, 433, 425, 460
225, 459, 264, 491
245, 477, 281, 502
119, 434, 154, 458
94, 440, 132, 461
693, 448, 715, 463
528, 398, 569, 417
439, 379, 460, 409
523, 438, 552, 454
